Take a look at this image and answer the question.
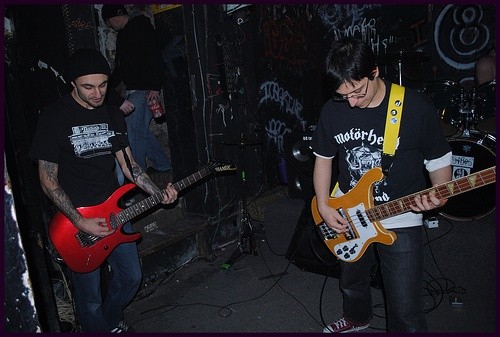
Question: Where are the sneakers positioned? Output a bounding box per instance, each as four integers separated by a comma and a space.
322, 315, 371, 333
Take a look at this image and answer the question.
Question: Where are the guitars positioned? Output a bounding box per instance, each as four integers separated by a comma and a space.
310, 162, 499, 265
49, 159, 239, 272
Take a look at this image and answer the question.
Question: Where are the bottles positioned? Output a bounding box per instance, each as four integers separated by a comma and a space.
215, 80, 223, 95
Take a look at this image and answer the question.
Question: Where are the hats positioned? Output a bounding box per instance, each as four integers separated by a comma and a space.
66, 48, 111, 81
102, 3, 129, 22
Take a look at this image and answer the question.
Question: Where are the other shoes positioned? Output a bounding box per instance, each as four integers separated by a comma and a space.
121, 189, 146, 207
108, 319, 135, 334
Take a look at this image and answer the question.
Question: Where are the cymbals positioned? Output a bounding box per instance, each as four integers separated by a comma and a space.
380, 50, 429, 57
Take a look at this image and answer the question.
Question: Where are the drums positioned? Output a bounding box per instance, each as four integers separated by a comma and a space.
428, 130, 497, 223
418, 80, 462, 136
472, 80, 498, 133
455, 92, 479, 133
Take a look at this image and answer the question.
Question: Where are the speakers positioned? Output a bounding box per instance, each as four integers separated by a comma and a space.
265, 195, 306, 255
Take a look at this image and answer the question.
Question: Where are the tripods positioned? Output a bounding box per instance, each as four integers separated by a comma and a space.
219, 144, 261, 270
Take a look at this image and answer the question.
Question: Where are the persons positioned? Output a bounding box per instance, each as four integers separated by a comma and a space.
308, 35, 454, 333
30, 47, 178, 334
101, 4, 171, 186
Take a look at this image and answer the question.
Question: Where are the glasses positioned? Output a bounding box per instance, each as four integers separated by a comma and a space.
333, 77, 370, 101
